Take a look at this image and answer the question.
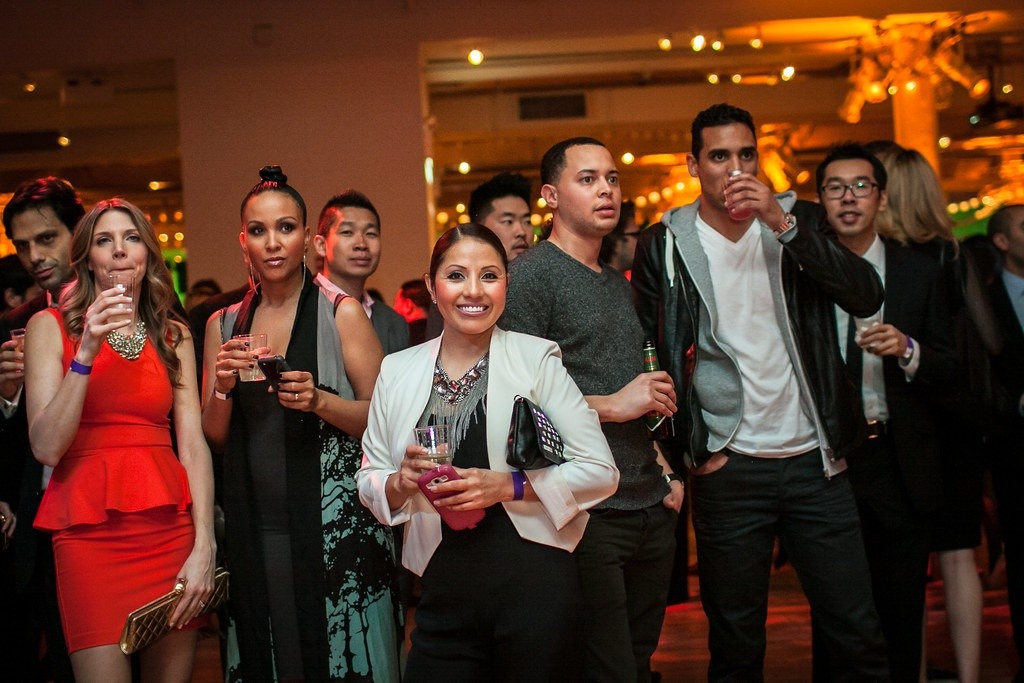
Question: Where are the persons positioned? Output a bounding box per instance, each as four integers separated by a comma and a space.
0, 172, 1002, 611
874, 143, 991, 683
630, 102, 886, 683
201, 165, 406, 683
499, 135, 684, 683
355, 222, 621, 683
314, 188, 411, 355
0, 177, 87, 683
815, 142, 971, 683
23, 197, 217, 683
985, 203, 1024, 417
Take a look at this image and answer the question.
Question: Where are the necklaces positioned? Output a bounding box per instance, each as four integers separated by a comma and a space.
107, 321, 147, 360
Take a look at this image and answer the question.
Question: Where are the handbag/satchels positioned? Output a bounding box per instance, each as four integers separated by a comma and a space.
119, 568, 230, 655
505, 395, 566, 469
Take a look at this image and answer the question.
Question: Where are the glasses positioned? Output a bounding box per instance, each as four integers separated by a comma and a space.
820, 181, 881, 199
617, 226, 644, 240
189, 290, 213, 297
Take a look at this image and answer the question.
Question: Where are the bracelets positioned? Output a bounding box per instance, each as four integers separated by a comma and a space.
903, 335, 913, 358
664, 473, 684, 487
213, 389, 231, 400
70, 360, 92, 375
512, 472, 527, 500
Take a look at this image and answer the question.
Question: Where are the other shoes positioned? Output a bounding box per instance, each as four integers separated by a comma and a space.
649, 671, 661, 683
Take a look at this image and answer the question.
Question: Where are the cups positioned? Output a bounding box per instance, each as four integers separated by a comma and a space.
854, 309, 882, 347
413, 425, 452, 474
101, 275, 135, 326
723, 171, 753, 221
11, 329, 26, 373
233, 334, 267, 381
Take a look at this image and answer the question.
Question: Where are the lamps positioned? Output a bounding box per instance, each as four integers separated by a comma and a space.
833, 17, 990, 123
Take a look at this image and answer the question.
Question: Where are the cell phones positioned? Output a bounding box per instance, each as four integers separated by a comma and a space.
419, 465, 486, 531
257, 355, 299, 393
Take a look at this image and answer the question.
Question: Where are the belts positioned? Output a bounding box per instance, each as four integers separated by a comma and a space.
868, 420, 889, 437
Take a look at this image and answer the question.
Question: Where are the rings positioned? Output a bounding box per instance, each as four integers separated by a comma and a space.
199, 600, 205, 607
296, 394, 299, 400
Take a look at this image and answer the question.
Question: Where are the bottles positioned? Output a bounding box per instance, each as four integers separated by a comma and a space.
643, 340, 674, 441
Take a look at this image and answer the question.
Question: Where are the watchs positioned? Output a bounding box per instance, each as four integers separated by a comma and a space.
773, 212, 794, 235
